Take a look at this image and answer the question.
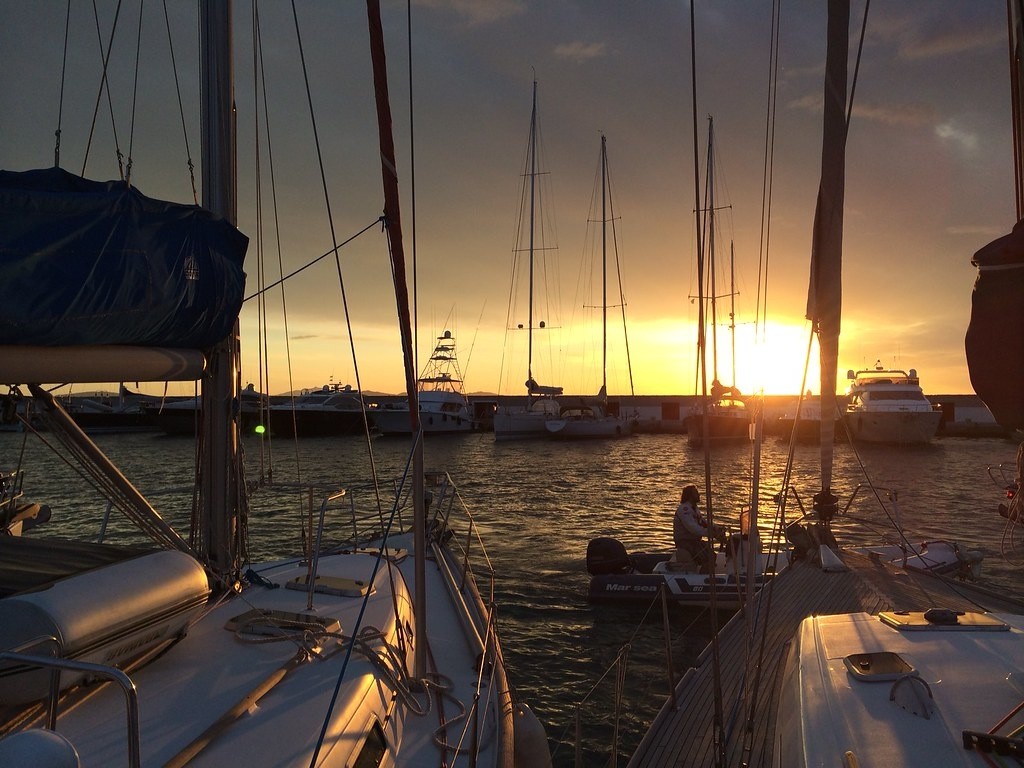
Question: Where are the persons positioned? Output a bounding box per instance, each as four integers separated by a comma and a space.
672, 485, 725, 574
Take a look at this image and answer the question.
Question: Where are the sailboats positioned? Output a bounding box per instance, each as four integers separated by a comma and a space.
2, 2, 1024, 766
